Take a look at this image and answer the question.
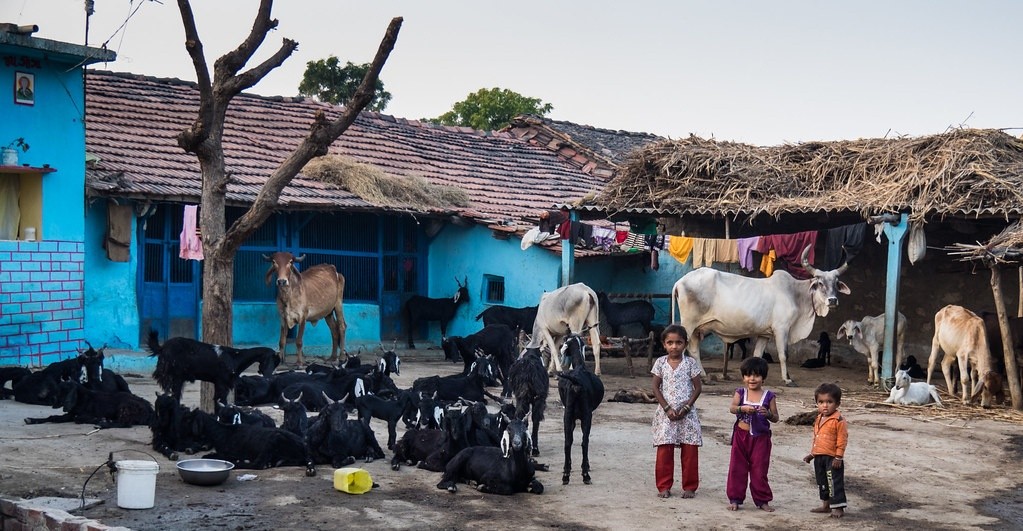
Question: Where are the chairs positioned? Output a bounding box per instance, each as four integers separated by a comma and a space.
577, 319, 656, 379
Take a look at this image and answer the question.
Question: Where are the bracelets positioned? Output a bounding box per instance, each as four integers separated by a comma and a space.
664, 406, 670, 412
835, 457, 843, 460
810, 453, 814, 457
683, 405, 690, 412
738, 406, 741, 413
770, 414, 774, 419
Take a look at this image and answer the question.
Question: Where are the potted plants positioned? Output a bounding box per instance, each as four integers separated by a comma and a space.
2, 138, 30, 166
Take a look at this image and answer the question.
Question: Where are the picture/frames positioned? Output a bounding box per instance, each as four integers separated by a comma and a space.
15, 71, 35, 104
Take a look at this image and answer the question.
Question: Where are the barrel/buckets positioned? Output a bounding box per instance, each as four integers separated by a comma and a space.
109, 449, 159, 509
334, 468, 373, 494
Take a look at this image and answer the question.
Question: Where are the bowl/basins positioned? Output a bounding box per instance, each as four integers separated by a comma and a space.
177, 460, 234, 485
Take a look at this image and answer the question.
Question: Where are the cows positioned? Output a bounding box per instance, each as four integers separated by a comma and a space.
518, 281, 602, 376
262, 252, 348, 369
672, 243, 851, 387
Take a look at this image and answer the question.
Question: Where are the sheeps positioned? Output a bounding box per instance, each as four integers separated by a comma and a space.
885, 366, 941, 406
597, 292, 750, 360
506, 339, 549, 456
817, 331, 832, 366
558, 321, 605, 485
405, 274, 469, 349
147, 330, 280, 414
437, 410, 544, 495
0, 340, 154, 428
153, 388, 385, 477
926, 304, 1006, 409
441, 304, 540, 388
901, 356, 922, 378
235, 337, 549, 472
837, 309, 908, 389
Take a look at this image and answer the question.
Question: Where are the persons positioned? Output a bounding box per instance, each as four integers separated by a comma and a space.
650, 326, 702, 498
803, 384, 848, 518
727, 357, 780, 511
